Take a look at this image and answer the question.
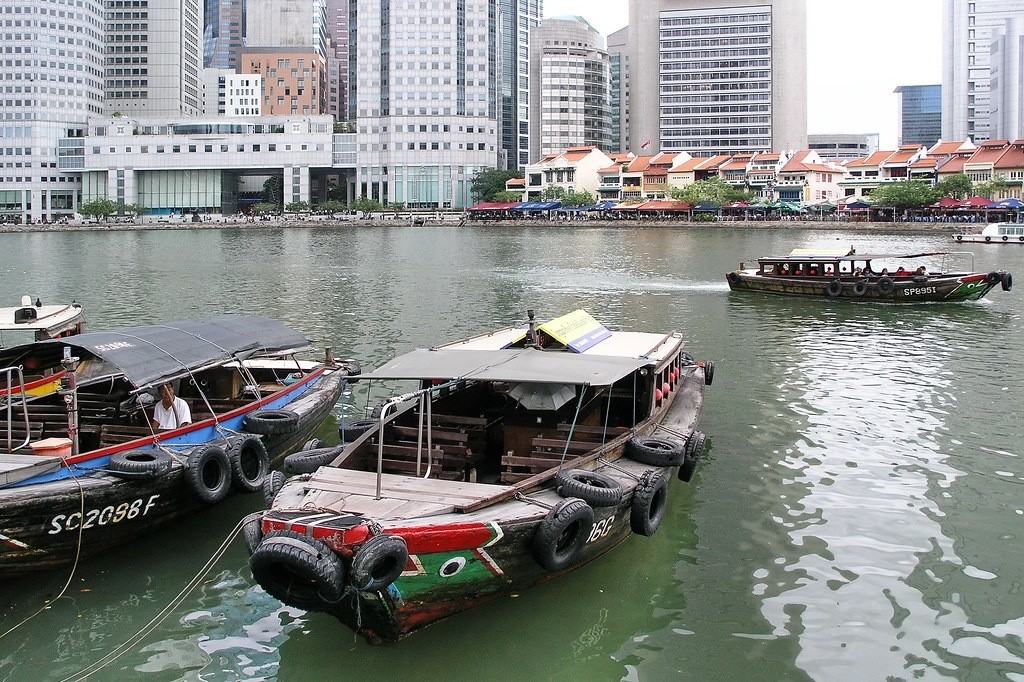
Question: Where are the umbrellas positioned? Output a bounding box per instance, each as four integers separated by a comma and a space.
750, 202, 808, 221
950, 196, 996, 221
986, 198, 1024, 223
922, 196, 959, 214
509, 383, 576, 410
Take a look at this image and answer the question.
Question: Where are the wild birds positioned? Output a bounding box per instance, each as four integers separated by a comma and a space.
71, 299, 82, 311
35, 297, 42, 310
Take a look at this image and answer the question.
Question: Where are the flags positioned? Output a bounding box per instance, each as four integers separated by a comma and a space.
641, 139, 650, 148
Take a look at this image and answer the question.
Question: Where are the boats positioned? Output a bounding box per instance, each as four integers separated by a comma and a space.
725, 246, 1013, 306
0, 296, 360, 564
953, 223, 1024, 243
237, 307, 714, 646
0, 303, 85, 349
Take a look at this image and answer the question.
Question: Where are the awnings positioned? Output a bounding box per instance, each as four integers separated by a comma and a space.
559, 201, 617, 212
468, 202, 562, 210
814, 201, 895, 222
611, 201, 750, 222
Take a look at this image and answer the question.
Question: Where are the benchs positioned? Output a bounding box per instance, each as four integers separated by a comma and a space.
0, 393, 255, 454
370, 408, 628, 486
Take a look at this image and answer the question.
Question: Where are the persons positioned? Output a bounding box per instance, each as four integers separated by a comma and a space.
914, 269, 924, 276
855, 267, 876, 277
152, 382, 192, 429
920, 266, 930, 275
896, 267, 907, 276
882, 268, 888, 275
470, 212, 1023, 222
